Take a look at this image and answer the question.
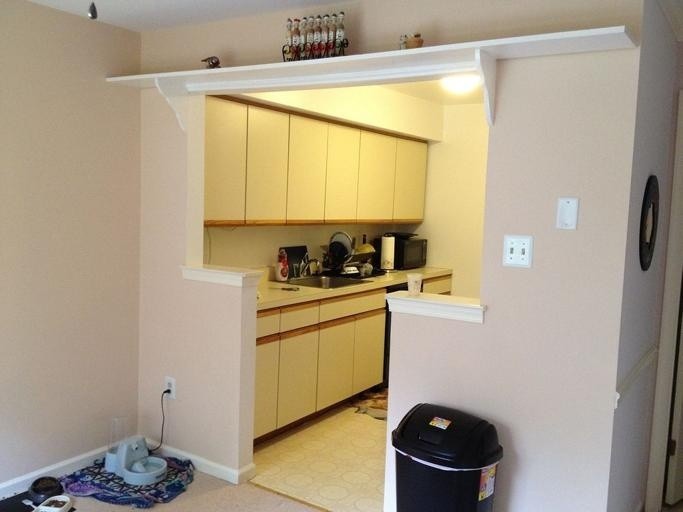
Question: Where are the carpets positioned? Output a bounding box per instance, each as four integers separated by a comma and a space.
61, 458, 193, 508
351, 389, 387, 422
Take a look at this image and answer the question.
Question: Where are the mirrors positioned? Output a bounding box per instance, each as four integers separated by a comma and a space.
636, 172, 659, 271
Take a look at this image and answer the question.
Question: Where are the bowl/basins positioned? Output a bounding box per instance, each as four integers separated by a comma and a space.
28, 475, 73, 511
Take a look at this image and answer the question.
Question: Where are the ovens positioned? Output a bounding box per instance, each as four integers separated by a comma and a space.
385, 282, 423, 383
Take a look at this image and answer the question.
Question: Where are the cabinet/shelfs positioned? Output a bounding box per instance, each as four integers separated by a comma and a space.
319, 289, 385, 412
252, 289, 318, 442
286, 109, 359, 227
423, 273, 453, 295
203, 86, 290, 221
356, 124, 427, 226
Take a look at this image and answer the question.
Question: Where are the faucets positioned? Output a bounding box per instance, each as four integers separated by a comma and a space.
299, 252, 322, 278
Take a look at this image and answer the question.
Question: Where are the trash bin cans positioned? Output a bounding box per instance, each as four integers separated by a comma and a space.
391, 402, 504, 512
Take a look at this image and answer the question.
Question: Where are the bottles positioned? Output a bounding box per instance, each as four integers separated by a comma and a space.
285, 11, 345, 58
400, 31, 423, 49
274, 249, 290, 281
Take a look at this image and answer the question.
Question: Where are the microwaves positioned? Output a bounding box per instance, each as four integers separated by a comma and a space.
374, 236, 428, 269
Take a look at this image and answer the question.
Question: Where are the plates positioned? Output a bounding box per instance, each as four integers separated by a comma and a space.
329, 231, 355, 263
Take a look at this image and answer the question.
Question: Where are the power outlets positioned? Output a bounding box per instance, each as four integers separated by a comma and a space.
163, 375, 176, 399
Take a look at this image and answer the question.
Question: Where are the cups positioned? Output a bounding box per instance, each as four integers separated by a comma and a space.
292, 260, 317, 279
320, 276, 330, 289
406, 273, 424, 297
